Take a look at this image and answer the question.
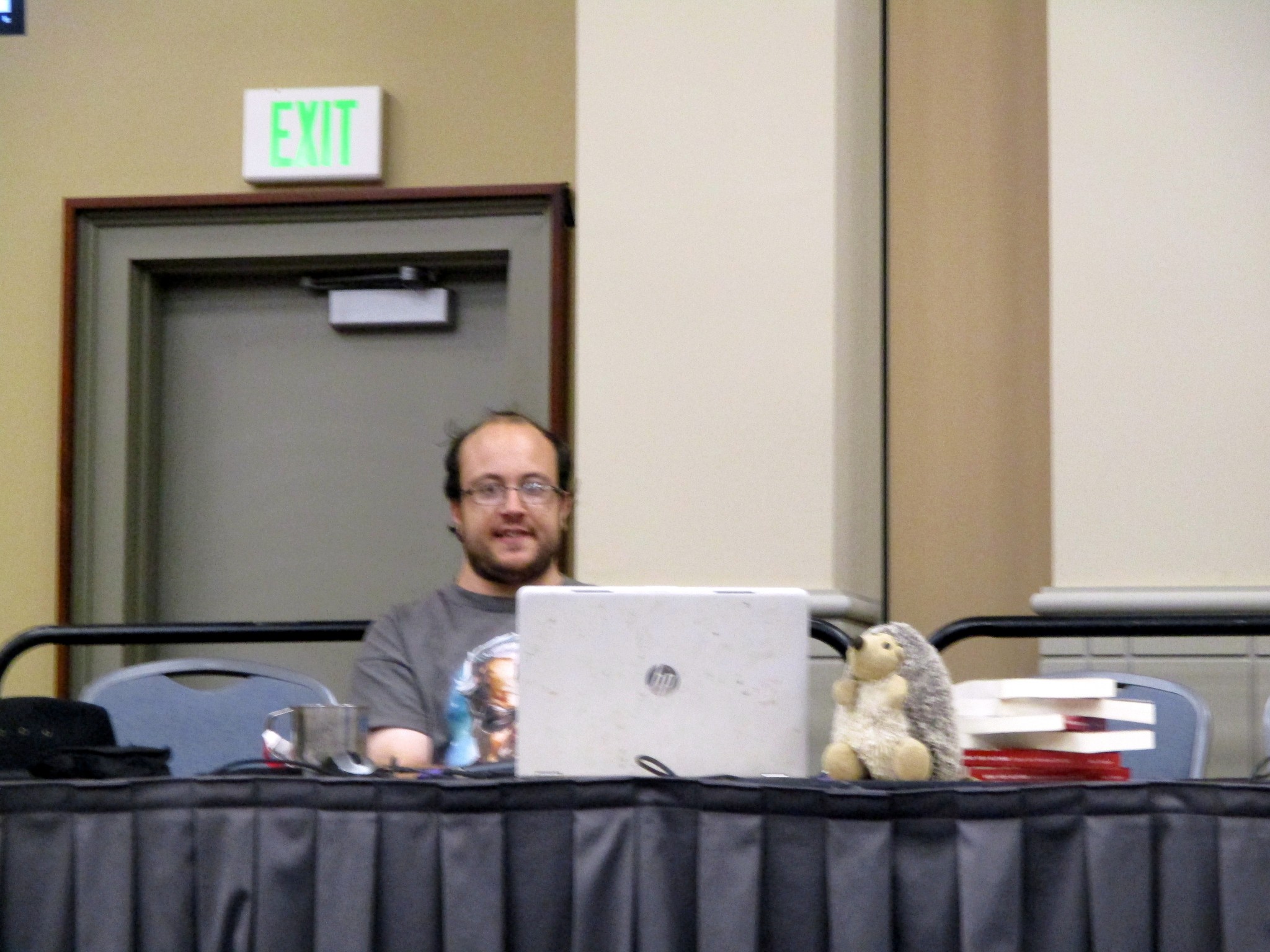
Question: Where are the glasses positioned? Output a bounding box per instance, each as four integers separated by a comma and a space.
457, 482, 566, 506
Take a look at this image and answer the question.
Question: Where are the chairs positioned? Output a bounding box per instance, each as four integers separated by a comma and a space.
1041, 671, 1210, 781
82, 656, 338, 774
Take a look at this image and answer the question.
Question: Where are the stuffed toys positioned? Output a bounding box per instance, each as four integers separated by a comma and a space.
819, 622, 968, 782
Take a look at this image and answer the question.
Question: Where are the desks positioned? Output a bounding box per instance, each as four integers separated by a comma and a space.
0, 776, 1270, 952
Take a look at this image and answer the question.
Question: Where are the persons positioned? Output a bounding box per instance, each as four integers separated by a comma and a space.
352, 410, 600, 783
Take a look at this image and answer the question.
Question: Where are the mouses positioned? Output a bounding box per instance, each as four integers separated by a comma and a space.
323, 748, 379, 779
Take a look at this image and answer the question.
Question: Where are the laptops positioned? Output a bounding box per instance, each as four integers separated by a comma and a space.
513, 586, 810, 780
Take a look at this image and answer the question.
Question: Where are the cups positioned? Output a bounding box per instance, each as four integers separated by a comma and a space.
266, 704, 371, 779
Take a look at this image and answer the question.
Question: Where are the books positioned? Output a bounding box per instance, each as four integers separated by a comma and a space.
951, 674, 1159, 785
